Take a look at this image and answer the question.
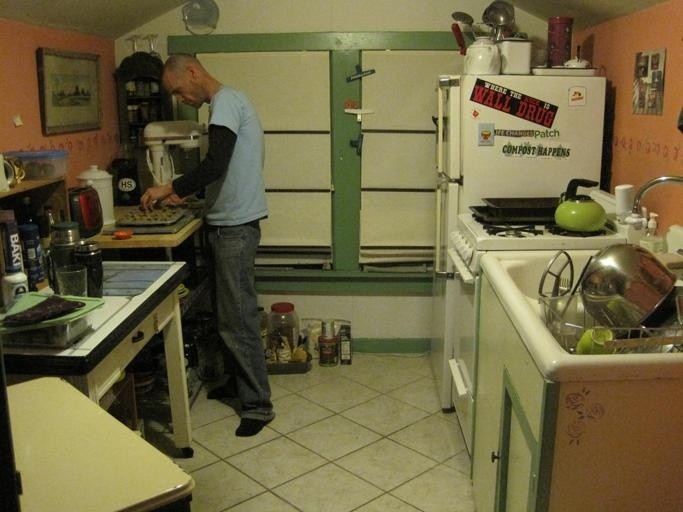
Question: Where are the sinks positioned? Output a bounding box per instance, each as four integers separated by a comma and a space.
480, 250, 682, 301
502, 300, 683, 383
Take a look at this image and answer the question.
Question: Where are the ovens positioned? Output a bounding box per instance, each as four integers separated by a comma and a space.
449, 248, 480, 457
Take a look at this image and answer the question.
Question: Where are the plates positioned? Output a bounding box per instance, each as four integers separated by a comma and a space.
532, 67, 602, 77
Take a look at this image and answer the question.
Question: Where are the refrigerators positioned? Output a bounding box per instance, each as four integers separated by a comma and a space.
428, 73, 609, 413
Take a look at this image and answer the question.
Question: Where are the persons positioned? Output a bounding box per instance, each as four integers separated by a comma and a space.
638, 56, 648, 76
140, 52, 276, 438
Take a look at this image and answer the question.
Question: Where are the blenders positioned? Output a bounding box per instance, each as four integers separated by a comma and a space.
142, 119, 208, 207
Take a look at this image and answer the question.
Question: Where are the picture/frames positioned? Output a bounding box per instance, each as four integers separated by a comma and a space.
38, 48, 101, 136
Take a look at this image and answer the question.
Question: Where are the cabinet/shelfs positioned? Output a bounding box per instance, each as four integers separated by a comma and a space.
471, 272, 560, 512
115, 52, 166, 143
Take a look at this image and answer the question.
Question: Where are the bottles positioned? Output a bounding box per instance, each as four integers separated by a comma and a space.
258, 307, 268, 351
75, 240, 104, 299
0, 209, 22, 273
50, 221, 82, 267
20, 224, 44, 290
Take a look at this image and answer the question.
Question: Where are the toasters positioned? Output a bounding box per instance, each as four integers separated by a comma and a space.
69, 186, 103, 239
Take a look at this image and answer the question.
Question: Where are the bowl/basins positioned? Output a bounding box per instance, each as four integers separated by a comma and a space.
577, 325, 616, 354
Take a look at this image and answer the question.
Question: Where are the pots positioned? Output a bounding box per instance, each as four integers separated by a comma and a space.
585, 246, 678, 336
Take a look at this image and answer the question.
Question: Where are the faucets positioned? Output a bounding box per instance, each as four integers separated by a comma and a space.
631, 176, 683, 211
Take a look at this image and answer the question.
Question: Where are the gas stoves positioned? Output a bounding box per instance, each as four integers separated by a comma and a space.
450, 189, 648, 254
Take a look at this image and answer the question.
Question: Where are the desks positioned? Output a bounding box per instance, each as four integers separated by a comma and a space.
1, 262, 194, 458
0, 376, 196, 512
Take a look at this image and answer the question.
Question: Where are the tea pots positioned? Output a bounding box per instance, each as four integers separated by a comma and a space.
553, 178, 608, 232
463, 39, 501, 75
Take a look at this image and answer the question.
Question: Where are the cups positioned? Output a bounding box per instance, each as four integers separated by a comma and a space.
55, 264, 88, 297
0, 154, 15, 195
